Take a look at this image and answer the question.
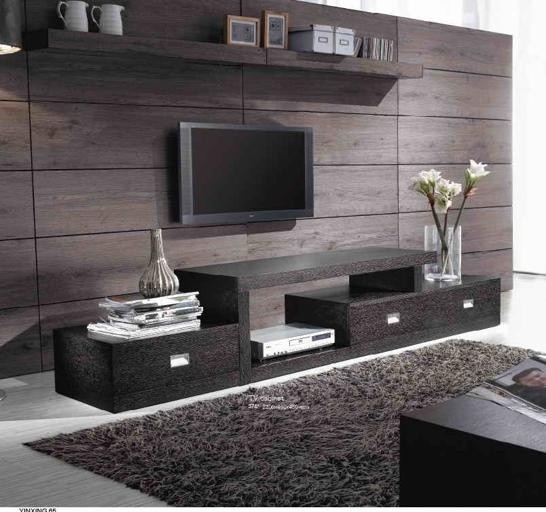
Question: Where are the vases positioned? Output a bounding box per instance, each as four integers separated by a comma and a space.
421, 223, 463, 282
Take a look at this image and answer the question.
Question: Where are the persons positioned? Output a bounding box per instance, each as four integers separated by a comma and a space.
512, 367, 546, 408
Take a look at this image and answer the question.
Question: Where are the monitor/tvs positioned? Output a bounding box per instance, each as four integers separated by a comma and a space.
178, 121, 314, 225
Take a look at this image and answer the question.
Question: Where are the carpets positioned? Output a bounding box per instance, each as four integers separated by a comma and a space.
20, 340, 546, 507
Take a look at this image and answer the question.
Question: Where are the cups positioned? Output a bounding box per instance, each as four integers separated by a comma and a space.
57, 0, 90, 33
92, 3, 127, 37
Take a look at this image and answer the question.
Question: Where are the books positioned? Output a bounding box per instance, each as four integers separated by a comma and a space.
354, 34, 394, 61
484, 356, 546, 410
85, 291, 204, 339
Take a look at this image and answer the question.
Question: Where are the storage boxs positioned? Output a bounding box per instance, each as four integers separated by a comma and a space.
288, 24, 356, 57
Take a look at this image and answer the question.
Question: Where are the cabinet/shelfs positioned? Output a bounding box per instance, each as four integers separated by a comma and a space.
51, 247, 501, 413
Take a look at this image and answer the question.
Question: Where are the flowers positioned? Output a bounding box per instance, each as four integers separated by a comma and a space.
408, 157, 491, 276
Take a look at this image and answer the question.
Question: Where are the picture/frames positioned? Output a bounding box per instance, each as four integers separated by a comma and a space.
224, 9, 290, 51
